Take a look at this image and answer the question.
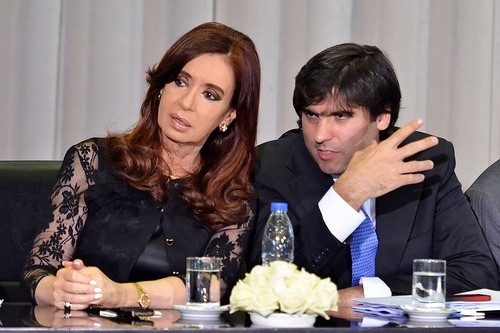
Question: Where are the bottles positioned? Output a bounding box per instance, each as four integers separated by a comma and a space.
261, 202, 295, 265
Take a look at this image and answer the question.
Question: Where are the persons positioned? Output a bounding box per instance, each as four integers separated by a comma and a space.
0, 305, 182, 330
249, 42, 500, 308
20, 21, 260, 313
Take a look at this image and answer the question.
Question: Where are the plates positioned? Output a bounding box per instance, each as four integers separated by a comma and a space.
174, 304, 231, 322
400, 306, 457, 323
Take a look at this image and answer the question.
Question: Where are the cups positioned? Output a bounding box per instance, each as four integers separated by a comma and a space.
185, 257, 222, 306
412, 259, 446, 312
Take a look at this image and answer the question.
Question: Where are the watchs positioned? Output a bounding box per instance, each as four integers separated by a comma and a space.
133, 280, 150, 310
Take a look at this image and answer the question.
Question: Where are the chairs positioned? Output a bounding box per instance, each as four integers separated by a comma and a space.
0, 161, 64, 300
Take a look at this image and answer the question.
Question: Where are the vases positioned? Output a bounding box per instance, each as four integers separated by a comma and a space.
246, 311, 319, 328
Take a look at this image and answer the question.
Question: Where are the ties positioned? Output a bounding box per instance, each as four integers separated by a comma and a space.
349, 206, 379, 329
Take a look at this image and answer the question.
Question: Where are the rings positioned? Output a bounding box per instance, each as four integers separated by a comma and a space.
62, 302, 70, 310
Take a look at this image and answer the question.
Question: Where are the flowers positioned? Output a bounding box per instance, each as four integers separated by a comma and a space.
228, 258, 339, 321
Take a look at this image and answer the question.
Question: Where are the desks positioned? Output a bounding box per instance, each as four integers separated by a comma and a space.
0, 297, 500, 333
